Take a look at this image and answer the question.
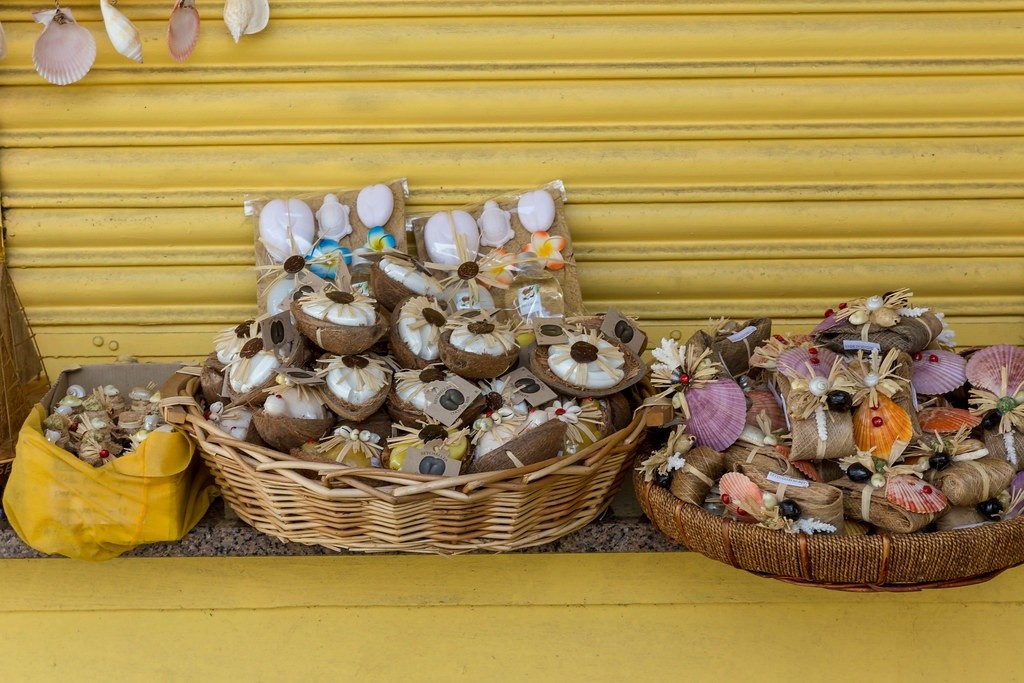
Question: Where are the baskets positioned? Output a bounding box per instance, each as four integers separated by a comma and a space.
634, 441, 1024, 585
157, 366, 658, 557
